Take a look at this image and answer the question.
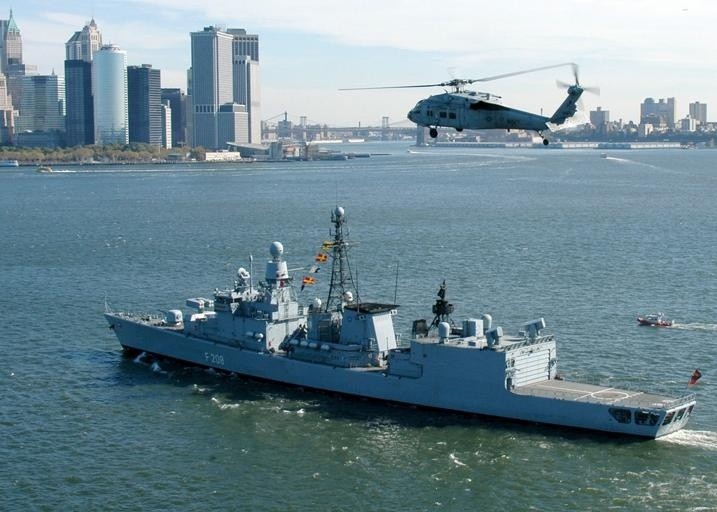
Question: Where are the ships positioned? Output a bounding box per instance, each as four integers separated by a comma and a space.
101, 177, 703, 440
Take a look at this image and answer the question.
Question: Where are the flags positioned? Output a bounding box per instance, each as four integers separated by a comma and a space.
301, 240, 335, 291
688, 370, 702, 387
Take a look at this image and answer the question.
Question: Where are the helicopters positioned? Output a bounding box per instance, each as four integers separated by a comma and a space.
339, 62, 602, 146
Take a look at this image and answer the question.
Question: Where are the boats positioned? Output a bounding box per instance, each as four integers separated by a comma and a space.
635, 311, 675, 327
405, 150, 411, 154
0, 159, 19, 168
598, 153, 606, 158
35, 166, 52, 174
